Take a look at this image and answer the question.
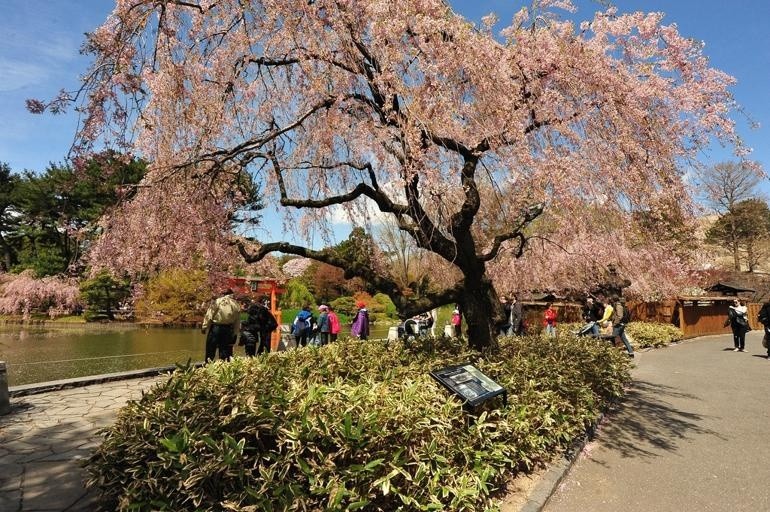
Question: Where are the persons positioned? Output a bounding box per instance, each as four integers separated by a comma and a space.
543, 302, 557, 338
597, 295, 615, 348
494, 290, 526, 337
581, 297, 599, 339
758, 298, 770, 360
200, 284, 464, 366
608, 295, 634, 358
722, 297, 749, 352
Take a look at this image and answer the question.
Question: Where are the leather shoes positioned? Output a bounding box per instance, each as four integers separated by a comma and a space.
628, 354, 633, 358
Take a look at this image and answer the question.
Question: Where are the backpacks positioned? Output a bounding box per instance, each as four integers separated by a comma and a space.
614, 304, 628, 323
295, 320, 306, 337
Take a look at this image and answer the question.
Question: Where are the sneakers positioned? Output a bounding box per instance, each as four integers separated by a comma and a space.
733, 348, 747, 352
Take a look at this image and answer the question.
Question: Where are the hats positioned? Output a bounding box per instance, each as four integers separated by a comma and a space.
318, 305, 327, 310
358, 302, 365, 308
